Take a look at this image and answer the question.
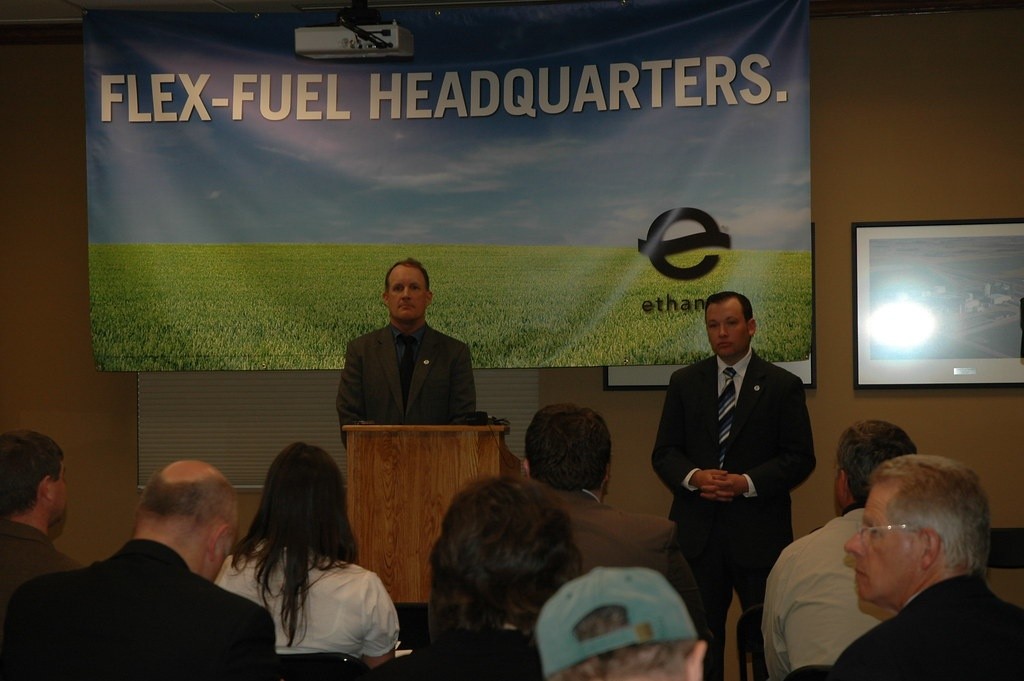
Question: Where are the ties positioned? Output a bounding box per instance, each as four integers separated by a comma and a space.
717, 367, 737, 470
397, 333, 416, 418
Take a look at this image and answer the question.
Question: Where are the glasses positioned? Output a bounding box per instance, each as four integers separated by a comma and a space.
851, 513, 949, 555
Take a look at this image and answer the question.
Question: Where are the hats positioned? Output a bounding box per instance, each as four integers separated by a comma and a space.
534, 565, 698, 679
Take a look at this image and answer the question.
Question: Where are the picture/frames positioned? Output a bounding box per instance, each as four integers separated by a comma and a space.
601, 222, 816, 392
851, 217, 1024, 389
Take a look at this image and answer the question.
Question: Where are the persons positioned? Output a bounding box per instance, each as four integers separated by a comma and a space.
0, 402, 1024, 681
651, 291, 816, 681
336, 258, 476, 450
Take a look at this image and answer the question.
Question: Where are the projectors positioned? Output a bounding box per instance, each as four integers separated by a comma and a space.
295, 25, 415, 58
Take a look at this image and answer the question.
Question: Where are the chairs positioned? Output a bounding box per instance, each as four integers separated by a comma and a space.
276, 652, 373, 681
736, 605, 770, 681
